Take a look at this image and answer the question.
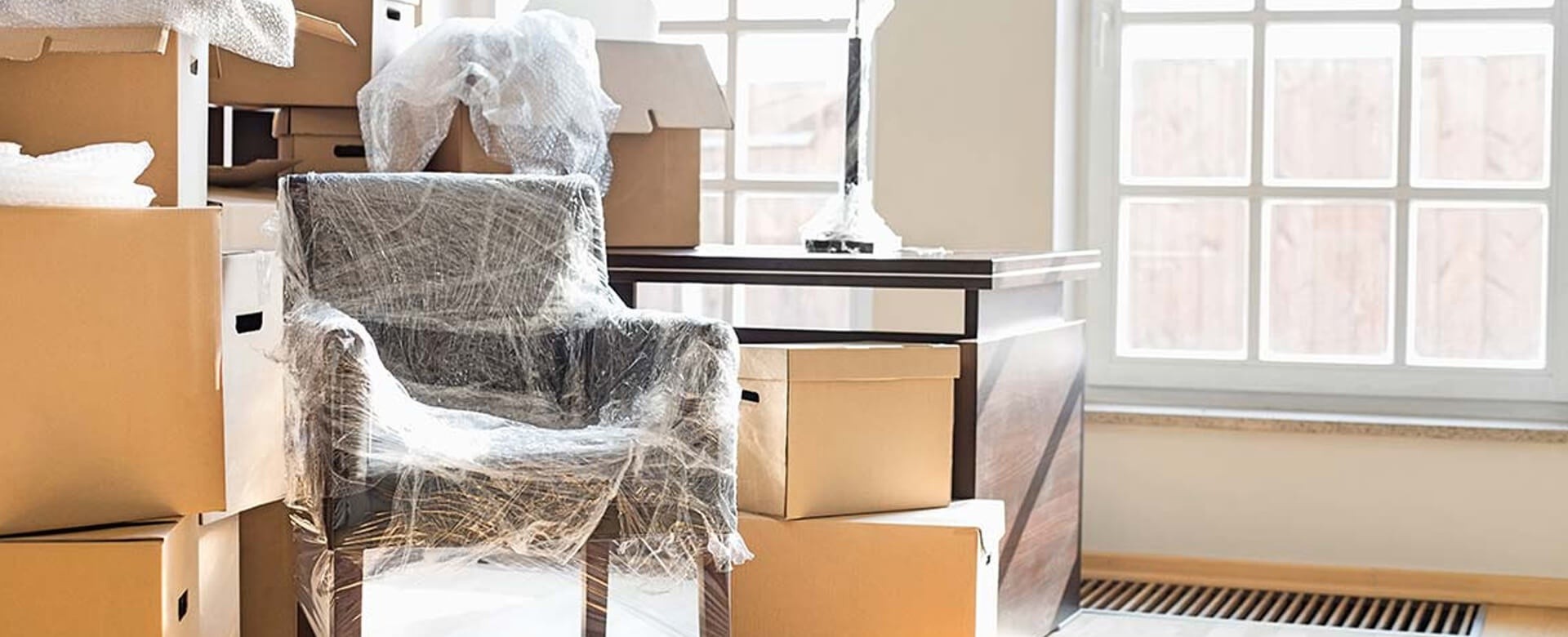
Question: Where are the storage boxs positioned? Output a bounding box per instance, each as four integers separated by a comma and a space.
731, 341, 960, 519
427, 35, 733, 248
1, 0, 415, 637
727, 498, 1005, 636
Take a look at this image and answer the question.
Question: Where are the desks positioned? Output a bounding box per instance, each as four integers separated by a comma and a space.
581, 242, 1103, 637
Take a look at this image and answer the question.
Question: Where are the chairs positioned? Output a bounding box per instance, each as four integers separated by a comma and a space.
279, 171, 740, 637
